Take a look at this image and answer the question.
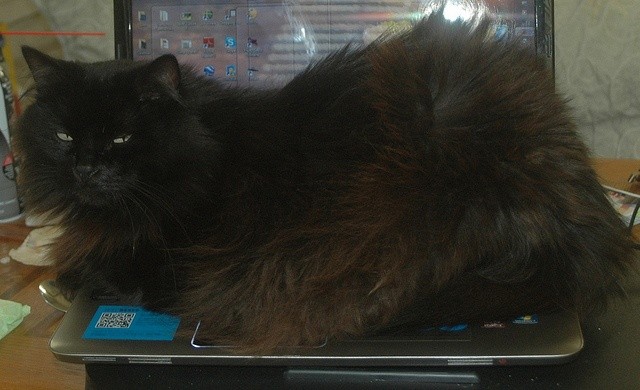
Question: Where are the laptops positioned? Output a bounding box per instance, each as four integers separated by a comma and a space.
46, 0, 584, 371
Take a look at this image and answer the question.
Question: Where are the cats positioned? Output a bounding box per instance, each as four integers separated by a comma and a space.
13, 0, 636, 356
